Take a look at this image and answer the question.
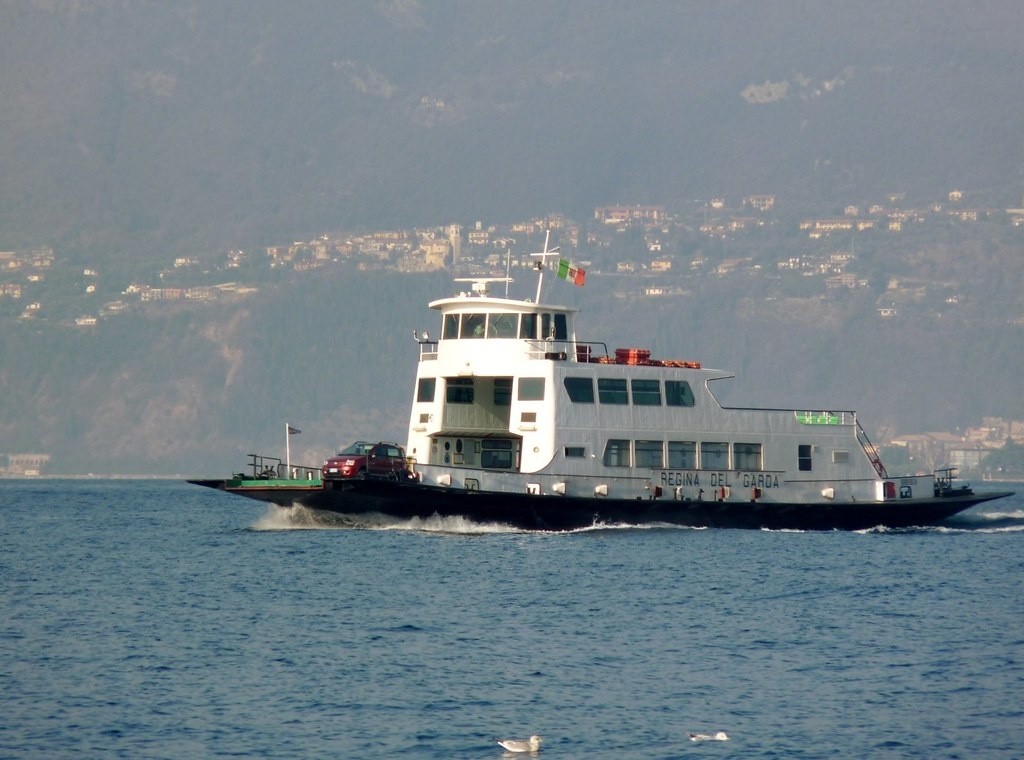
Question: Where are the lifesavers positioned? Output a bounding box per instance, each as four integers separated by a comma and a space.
650, 359, 701, 369
597, 356, 616, 364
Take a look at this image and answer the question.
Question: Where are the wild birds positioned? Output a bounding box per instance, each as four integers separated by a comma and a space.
685, 731, 731, 742
493, 735, 544, 753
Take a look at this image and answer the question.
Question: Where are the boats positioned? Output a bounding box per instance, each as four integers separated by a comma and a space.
185, 231, 1018, 530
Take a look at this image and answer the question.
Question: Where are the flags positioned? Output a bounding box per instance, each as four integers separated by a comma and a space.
556, 258, 585, 286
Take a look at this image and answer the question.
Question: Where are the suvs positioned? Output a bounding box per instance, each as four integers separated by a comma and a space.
321, 440, 407, 479
